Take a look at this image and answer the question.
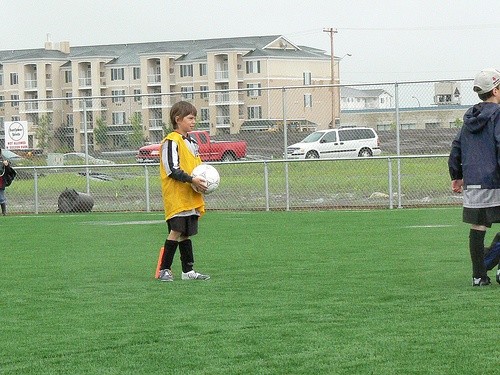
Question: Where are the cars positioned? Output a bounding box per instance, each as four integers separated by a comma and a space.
45, 152, 116, 170
1, 149, 42, 174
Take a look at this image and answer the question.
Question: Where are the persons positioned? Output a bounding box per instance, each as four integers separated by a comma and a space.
158, 100, 210, 281
0, 149, 17, 215
448, 69, 500, 286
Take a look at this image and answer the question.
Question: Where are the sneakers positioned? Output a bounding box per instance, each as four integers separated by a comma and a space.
496, 270, 500, 283
472, 276, 492, 286
181, 271, 210, 281
158, 270, 173, 281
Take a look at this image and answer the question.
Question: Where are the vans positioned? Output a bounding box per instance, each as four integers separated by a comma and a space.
282, 127, 382, 160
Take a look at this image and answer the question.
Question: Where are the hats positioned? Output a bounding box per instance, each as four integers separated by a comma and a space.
473, 68, 500, 94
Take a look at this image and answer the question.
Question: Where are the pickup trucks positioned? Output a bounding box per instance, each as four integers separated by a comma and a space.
135, 130, 247, 169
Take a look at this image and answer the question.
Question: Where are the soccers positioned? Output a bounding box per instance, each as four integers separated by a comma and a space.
190, 164, 221, 195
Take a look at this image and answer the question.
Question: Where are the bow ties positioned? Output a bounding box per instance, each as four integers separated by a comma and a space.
182, 136, 192, 143
